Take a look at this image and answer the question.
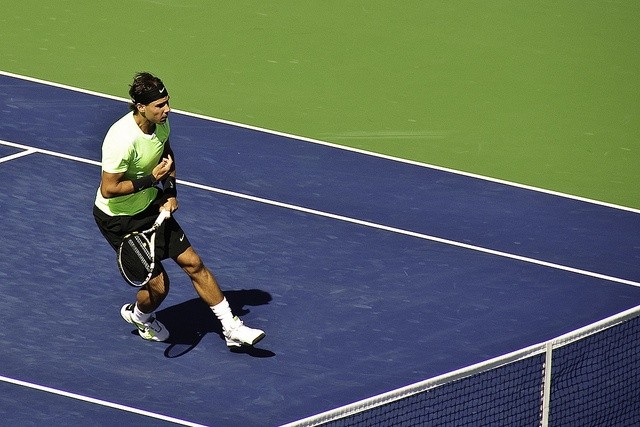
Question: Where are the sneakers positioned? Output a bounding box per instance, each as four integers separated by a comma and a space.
222, 316, 266, 348
120, 302, 170, 342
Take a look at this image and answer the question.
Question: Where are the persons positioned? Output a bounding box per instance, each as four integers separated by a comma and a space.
92, 72, 266, 350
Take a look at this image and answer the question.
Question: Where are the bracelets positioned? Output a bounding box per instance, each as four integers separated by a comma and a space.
131, 174, 157, 192
161, 176, 177, 200
